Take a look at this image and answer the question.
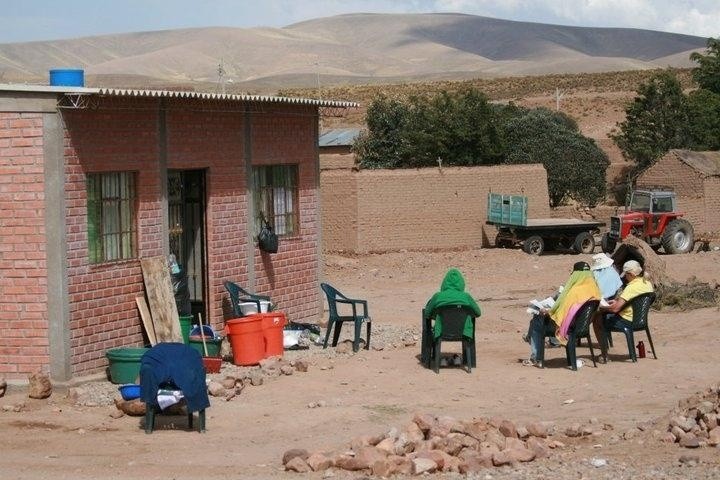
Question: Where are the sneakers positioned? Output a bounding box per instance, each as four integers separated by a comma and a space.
596, 354, 607, 364
522, 332, 531, 344
544, 340, 560, 348
522, 357, 542, 367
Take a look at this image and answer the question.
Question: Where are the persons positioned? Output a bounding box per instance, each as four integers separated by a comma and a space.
424, 268, 481, 359
522, 261, 600, 368
593, 260, 653, 364
544, 253, 623, 348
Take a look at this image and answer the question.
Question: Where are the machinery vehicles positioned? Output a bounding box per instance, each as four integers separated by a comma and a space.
602, 190, 694, 255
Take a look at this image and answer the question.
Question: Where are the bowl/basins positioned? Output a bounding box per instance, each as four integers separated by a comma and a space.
104, 348, 151, 383
239, 302, 271, 316
202, 356, 223, 373
118, 385, 141, 400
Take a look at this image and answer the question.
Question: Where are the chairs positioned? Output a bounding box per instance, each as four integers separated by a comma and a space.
422, 305, 476, 373
321, 282, 372, 352
139, 342, 211, 433
536, 299, 600, 371
224, 280, 271, 319
578, 286, 629, 361
601, 292, 657, 361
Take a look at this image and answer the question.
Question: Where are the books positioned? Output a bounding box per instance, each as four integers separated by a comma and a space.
526, 296, 556, 315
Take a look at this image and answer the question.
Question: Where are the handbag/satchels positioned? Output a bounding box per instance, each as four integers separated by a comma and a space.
258, 222, 278, 253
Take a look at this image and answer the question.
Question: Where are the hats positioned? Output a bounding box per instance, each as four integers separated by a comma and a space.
570, 261, 590, 275
591, 252, 615, 270
619, 260, 640, 278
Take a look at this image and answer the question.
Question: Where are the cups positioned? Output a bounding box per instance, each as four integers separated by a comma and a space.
576, 360, 585, 367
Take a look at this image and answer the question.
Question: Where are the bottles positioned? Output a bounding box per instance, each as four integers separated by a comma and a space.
638, 341, 646, 358
